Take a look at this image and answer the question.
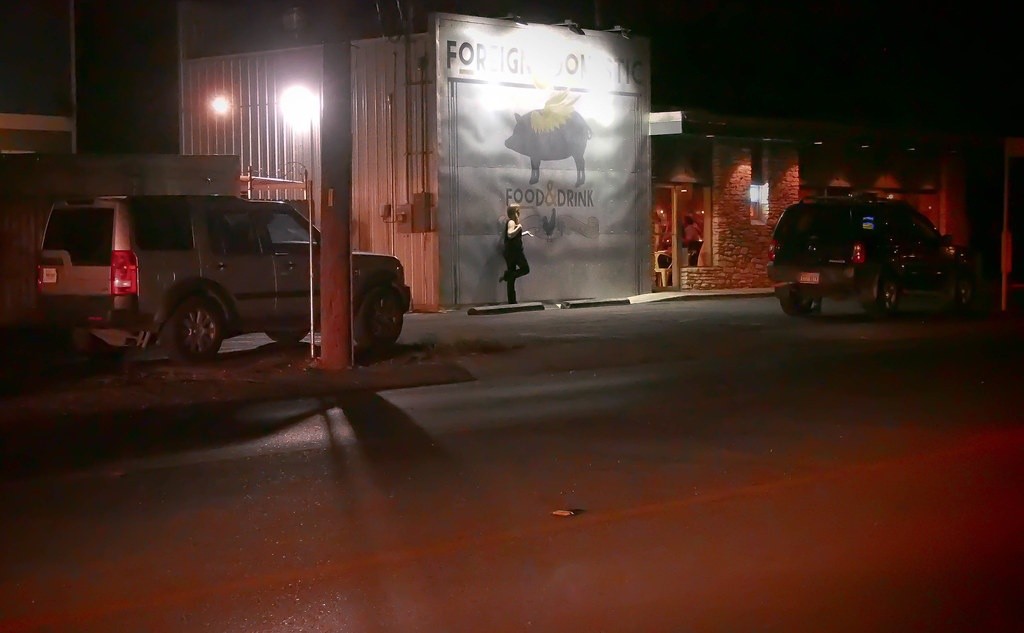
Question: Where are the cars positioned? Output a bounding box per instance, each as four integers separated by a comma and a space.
764, 195, 979, 312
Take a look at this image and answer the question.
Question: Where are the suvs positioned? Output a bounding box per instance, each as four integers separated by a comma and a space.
34, 193, 411, 367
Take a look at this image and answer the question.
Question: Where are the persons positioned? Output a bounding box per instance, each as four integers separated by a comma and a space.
502, 203, 529, 305
